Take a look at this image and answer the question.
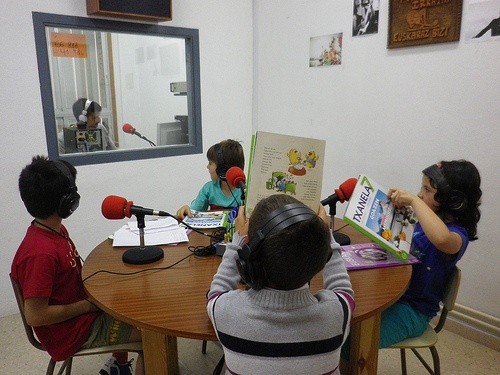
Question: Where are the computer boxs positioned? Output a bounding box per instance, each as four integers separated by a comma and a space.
63, 127, 103, 154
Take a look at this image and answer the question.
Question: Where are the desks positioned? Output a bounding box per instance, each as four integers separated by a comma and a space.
81, 213, 412, 375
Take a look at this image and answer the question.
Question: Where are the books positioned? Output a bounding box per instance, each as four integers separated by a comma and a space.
244, 131, 324, 218
338, 175, 419, 270
170, 209, 233, 246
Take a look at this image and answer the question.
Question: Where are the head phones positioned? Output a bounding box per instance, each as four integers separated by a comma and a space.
422, 164, 465, 213
236, 202, 335, 291
53, 160, 80, 219
78, 99, 93, 123
214, 144, 229, 181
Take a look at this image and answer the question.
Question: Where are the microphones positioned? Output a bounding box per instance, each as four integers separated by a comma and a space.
226, 166, 247, 189
122, 123, 146, 139
101, 195, 169, 220
322, 178, 358, 207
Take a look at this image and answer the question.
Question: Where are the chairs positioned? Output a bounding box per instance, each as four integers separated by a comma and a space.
378, 267, 461, 375
9, 274, 145, 375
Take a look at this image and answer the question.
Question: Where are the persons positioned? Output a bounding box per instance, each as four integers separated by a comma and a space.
10, 155, 147, 375
58, 99, 119, 154
177, 139, 247, 218
205, 194, 354, 374
340, 160, 483, 375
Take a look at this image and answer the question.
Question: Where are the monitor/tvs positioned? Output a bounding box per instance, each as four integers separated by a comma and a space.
156, 122, 188, 146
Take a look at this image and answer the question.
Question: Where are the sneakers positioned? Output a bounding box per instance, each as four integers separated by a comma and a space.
97, 357, 135, 375
